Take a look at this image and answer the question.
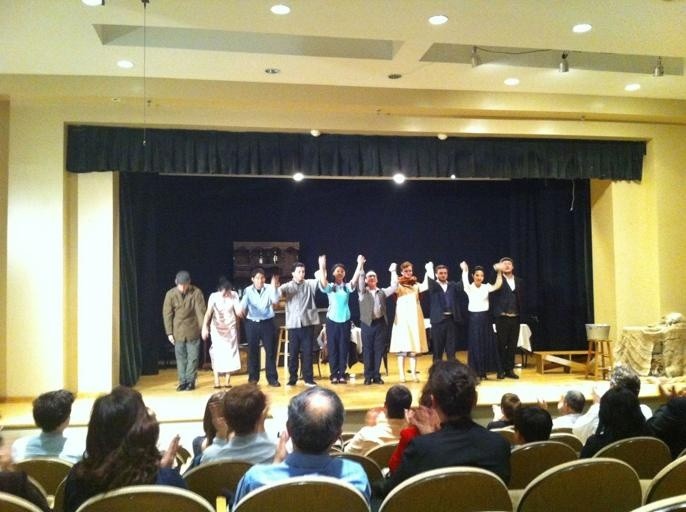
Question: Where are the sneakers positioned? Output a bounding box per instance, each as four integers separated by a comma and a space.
496, 368, 520, 380
362, 376, 385, 385
213, 382, 234, 391
268, 378, 281, 388
286, 379, 296, 388
398, 375, 421, 385
330, 375, 348, 385
177, 382, 195, 392
302, 378, 318, 388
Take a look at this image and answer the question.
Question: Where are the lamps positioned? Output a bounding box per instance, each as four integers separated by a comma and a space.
559, 53, 568, 72
471, 45, 482, 67
653, 56, 663, 76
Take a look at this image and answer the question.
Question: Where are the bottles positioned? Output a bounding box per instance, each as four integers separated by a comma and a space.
348, 374, 357, 386
272, 250, 280, 263
258, 249, 264, 263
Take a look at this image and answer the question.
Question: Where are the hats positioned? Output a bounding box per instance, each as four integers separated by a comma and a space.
175, 270, 191, 286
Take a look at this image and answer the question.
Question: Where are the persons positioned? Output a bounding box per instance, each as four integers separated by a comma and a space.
11, 360, 686, 512
163, 256, 527, 388
